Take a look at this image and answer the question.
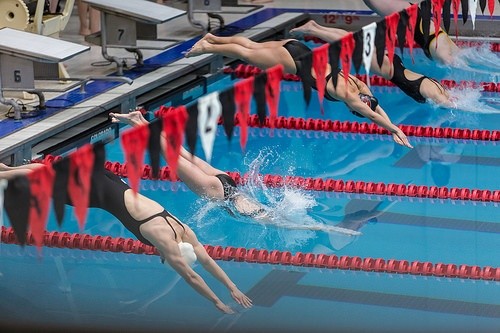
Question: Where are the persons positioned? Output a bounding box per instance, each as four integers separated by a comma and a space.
43, 0, 167, 35
110, 110, 362, 237
187, 32, 417, 149
0, 158, 254, 316
361, 0, 464, 68
291, 20, 462, 110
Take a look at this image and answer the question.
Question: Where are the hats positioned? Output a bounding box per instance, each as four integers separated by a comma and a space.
164, 242, 196, 273
352, 93, 378, 118
